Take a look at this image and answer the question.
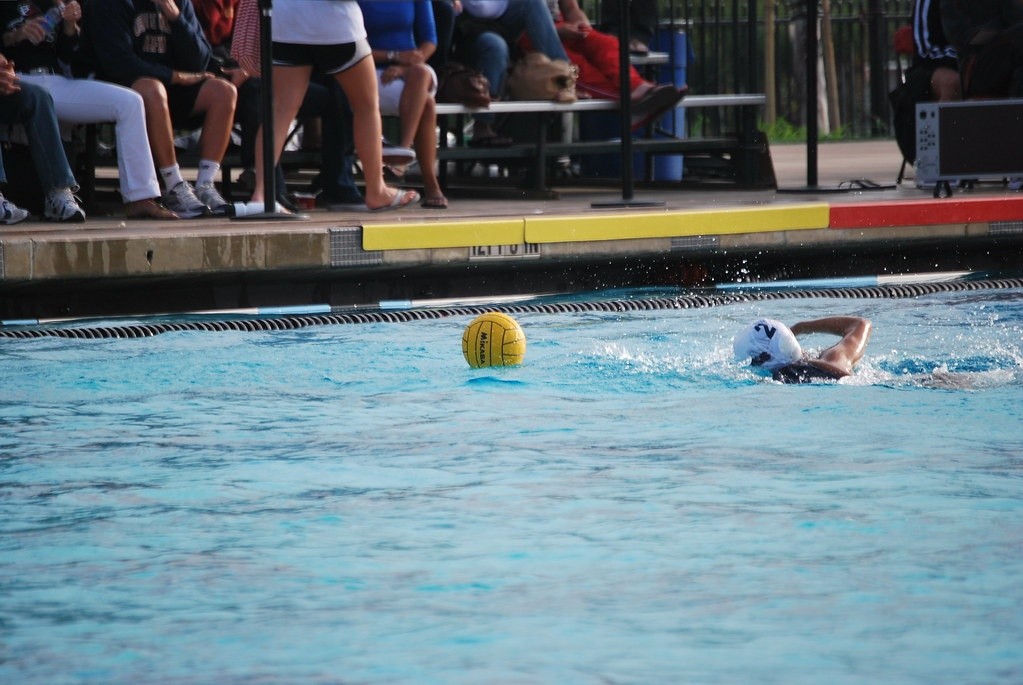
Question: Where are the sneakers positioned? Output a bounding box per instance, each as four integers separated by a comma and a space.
122, 199, 181, 219
0, 193, 31, 225
161, 181, 212, 219
39, 185, 86, 222
194, 183, 232, 215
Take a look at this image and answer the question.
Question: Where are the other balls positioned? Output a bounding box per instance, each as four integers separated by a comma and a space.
460, 313, 527, 368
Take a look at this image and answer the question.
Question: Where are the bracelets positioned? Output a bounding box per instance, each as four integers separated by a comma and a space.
395, 51, 400, 64
387, 49, 393, 65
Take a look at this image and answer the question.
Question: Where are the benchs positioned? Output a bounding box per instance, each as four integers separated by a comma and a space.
338, 94, 770, 200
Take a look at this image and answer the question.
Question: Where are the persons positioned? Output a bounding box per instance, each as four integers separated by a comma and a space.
789, 0, 830, 138
913, 0, 1023, 193
0, 0, 688, 225
733, 316, 873, 384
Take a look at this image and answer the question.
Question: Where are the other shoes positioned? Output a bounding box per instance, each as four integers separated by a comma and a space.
353, 134, 416, 166
630, 83, 688, 118
423, 183, 448, 208
466, 135, 514, 146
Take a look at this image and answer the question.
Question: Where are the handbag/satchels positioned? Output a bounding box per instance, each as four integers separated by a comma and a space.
889, 50, 933, 166
207, 54, 240, 80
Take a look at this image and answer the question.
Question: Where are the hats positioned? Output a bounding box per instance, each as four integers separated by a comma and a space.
731, 317, 803, 378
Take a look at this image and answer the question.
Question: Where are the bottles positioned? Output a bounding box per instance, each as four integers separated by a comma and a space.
29, 4, 66, 46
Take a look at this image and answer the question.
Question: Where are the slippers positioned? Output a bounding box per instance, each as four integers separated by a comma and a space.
385, 161, 407, 179
280, 207, 306, 220
365, 189, 421, 213
